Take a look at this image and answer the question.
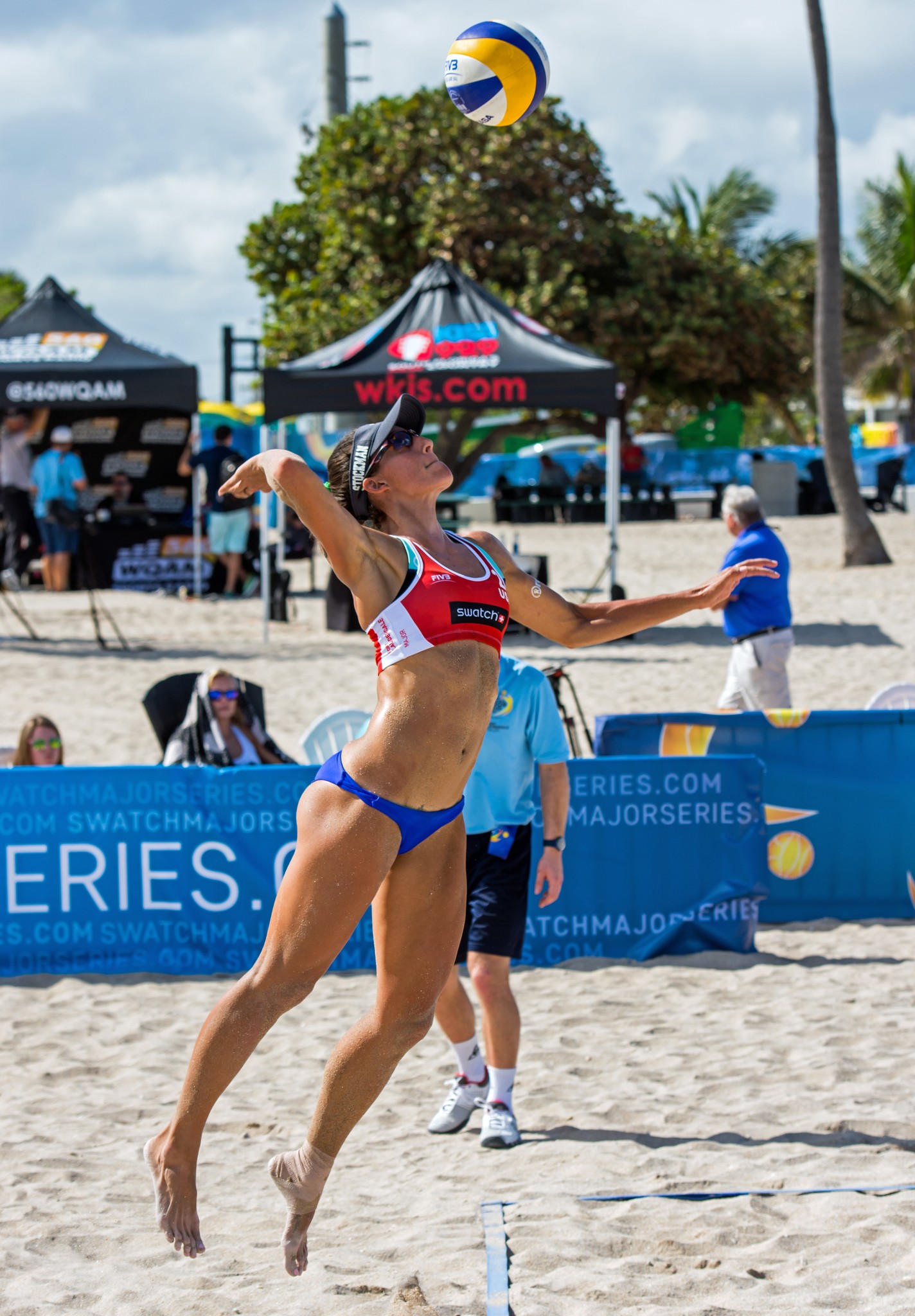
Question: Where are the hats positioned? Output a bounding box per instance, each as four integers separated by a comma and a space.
49, 425, 74, 444
349, 394, 427, 519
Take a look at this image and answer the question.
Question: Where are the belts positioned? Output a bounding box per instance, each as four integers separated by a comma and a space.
732, 623, 787, 646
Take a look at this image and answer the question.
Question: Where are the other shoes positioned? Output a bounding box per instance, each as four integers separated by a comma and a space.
240, 574, 259, 597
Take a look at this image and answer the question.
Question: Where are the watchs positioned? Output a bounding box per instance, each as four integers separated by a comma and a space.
543, 835, 566, 852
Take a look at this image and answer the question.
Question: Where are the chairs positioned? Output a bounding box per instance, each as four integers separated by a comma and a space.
140, 667, 371, 771
861, 455, 908, 515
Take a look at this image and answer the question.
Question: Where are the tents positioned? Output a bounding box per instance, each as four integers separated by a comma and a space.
443, 439, 915, 499
0, 274, 202, 597
262, 257, 624, 643
199, 402, 353, 565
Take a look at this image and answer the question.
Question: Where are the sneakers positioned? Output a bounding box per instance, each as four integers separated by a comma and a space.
473, 1096, 523, 1149
427, 1063, 491, 1134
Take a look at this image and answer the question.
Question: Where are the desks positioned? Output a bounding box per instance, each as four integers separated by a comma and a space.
73, 507, 183, 593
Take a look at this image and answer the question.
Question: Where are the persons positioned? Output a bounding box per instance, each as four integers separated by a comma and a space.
176, 423, 259, 600
145, 394, 779, 1278
0, 404, 153, 593
430, 652, 573, 1149
710, 484, 795, 709
493, 434, 657, 521
162, 670, 295, 765
12, 715, 63, 766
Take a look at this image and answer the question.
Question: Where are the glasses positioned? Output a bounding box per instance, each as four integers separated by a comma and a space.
29, 738, 62, 751
207, 689, 240, 702
357, 427, 419, 500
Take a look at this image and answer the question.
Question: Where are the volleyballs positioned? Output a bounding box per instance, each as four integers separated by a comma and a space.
445, 19, 550, 127
